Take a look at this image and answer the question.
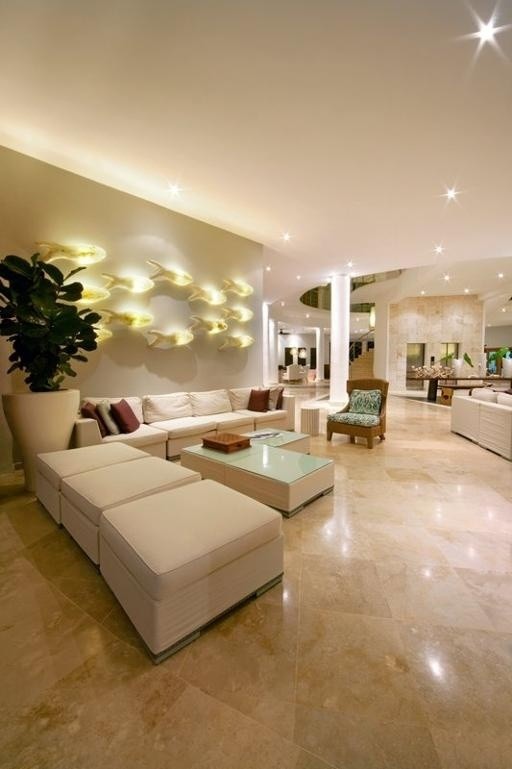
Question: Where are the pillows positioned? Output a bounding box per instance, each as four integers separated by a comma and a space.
348, 389, 382, 416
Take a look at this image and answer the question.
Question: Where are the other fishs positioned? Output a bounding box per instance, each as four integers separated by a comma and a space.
32, 241, 255, 350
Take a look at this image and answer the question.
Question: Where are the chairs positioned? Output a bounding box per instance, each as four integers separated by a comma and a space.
448, 388, 511, 461
326, 378, 390, 449
70, 388, 296, 459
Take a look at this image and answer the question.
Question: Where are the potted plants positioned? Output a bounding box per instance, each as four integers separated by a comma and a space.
1, 249, 101, 492
